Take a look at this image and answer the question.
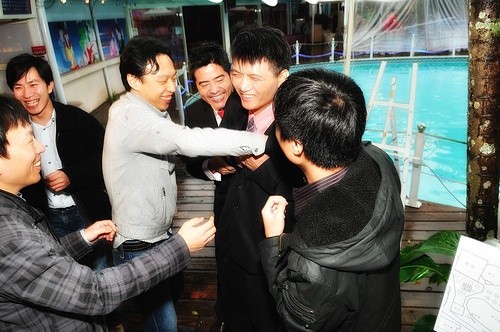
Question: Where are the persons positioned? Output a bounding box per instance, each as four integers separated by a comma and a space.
261, 67, 405, 332
383, 9, 403, 56
0, 92, 217, 331
360, 1, 379, 30
215, 27, 314, 331
5, 52, 123, 331
301, 16, 314, 51
101, 35, 272, 331
182, 41, 248, 229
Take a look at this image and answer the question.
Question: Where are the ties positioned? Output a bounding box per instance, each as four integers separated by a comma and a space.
247, 118, 257, 133
